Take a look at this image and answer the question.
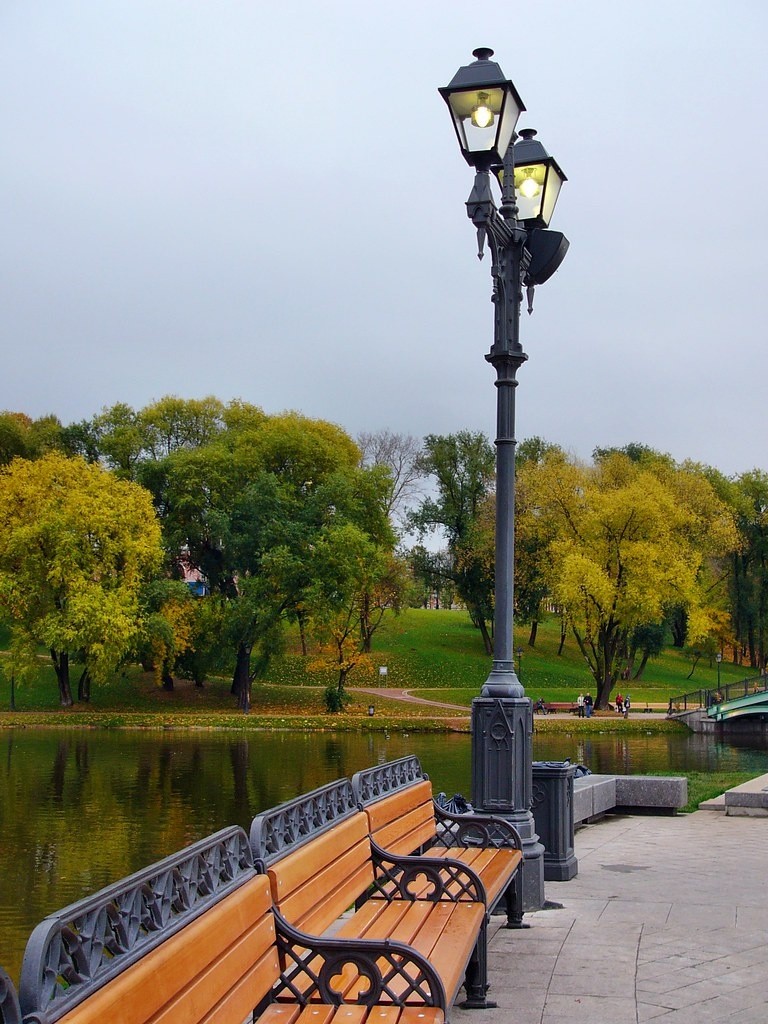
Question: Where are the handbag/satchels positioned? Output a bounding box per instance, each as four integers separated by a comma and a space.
622, 707, 627, 712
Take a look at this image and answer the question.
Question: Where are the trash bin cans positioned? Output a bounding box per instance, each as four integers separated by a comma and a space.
530, 759, 580, 882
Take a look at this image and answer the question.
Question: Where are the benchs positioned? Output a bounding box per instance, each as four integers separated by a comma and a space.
352, 752, 545, 992
25, 823, 449, 1024
249, 773, 503, 1020
533, 702, 580, 715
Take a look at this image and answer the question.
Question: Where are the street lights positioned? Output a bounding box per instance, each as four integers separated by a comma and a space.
439, 46, 569, 917
516, 647, 524, 683
716, 654, 722, 692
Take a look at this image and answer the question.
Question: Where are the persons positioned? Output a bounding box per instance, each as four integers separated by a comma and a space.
624, 695, 630, 712
536, 697, 546, 715
577, 692, 593, 718
616, 693, 623, 712
